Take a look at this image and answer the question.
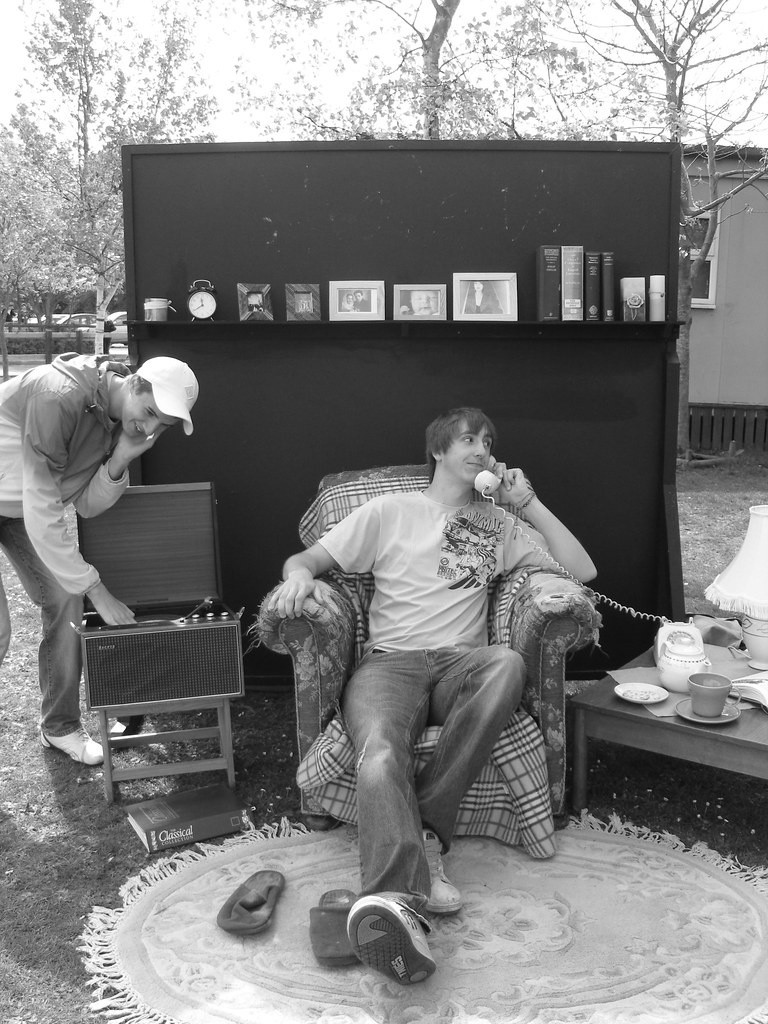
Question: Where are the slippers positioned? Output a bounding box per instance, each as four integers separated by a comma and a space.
309, 889, 358, 968
216, 870, 285, 936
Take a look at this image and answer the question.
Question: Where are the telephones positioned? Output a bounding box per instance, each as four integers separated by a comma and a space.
474, 456, 502, 495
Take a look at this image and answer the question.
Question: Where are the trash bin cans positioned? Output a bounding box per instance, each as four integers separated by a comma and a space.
103, 337, 111, 354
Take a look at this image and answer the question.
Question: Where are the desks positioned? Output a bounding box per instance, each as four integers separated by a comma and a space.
98, 699, 234, 803
570, 617, 767, 814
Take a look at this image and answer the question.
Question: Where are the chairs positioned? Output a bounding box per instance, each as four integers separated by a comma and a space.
256, 464, 603, 858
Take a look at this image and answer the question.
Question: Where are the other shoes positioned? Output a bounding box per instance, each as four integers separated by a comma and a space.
41, 724, 103, 766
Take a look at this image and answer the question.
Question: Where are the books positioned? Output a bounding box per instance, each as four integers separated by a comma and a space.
537, 246, 615, 322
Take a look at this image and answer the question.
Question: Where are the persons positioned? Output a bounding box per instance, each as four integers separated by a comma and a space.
268, 406, 597, 985
340, 290, 370, 312
464, 281, 496, 313
400, 291, 438, 316
0, 350, 200, 767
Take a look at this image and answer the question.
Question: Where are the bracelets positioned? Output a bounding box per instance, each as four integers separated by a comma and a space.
512, 492, 536, 512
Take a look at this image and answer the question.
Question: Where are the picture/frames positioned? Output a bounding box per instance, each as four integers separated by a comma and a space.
452, 272, 519, 321
329, 281, 386, 321
392, 284, 447, 320
237, 283, 274, 321
285, 283, 321, 321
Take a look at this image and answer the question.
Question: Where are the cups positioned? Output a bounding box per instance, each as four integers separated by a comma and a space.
687, 671, 743, 718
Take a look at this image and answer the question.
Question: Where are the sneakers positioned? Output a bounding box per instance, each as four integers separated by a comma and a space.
418, 829, 463, 912
347, 894, 437, 985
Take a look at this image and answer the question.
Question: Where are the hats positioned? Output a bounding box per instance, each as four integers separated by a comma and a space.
136, 356, 200, 436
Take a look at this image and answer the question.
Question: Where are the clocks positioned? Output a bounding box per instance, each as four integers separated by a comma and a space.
186, 279, 217, 321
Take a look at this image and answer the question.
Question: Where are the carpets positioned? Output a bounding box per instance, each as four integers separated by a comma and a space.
78, 811, 767, 1024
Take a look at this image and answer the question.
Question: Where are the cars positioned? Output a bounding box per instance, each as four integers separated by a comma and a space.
13, 311, 128, 342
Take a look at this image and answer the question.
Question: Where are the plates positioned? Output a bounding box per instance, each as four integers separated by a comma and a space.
613, 682, 669, 704
674, 697, 742, 724
